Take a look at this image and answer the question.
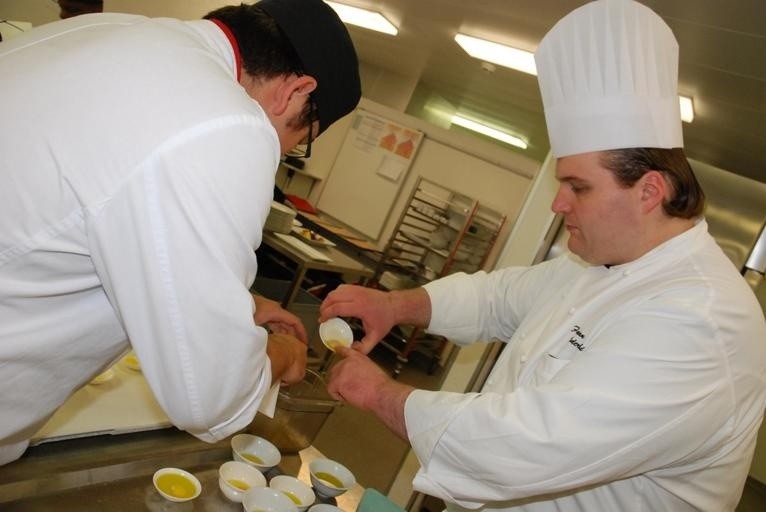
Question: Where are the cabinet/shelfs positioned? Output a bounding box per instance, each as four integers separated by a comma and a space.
259, 162, 506, 379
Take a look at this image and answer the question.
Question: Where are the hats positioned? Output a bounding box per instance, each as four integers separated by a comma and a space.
251, 1, 364, 138
533, 0, 688, 160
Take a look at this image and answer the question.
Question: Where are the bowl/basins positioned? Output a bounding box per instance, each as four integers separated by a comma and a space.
231, 433, 282, 473
308, 459, 357, 497
270, 474, 316, 512
241, 487, 299, 512
218, 461, 266, 503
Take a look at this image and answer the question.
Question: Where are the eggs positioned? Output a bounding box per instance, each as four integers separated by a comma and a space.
156, 473, 196, 499
327, 339, 346, 350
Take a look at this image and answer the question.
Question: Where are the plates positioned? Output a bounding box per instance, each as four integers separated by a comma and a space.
264, 199, 297, 235
291, 227, 336, 247
319, 317, 354, 353
152, 466, 202, 502
274, 233, 333, 262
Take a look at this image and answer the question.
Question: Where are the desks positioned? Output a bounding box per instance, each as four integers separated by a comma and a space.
0, 428, 364, 512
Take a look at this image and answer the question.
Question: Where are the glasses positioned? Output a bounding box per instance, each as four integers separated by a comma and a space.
285, 66, 315, 160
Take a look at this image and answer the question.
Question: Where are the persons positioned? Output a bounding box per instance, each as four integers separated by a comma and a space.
0, 2, 361, 472
320, 3, 764, 509
